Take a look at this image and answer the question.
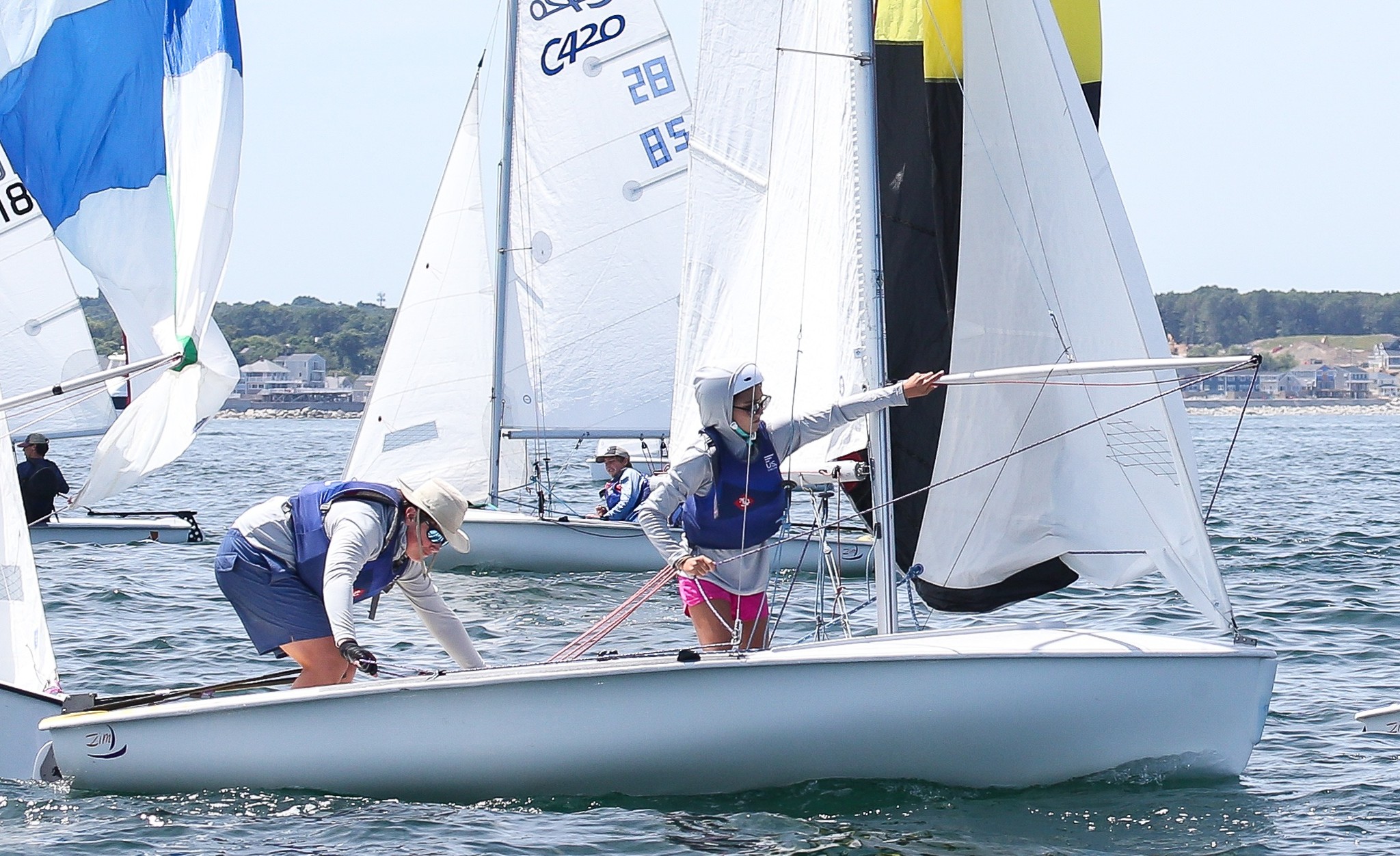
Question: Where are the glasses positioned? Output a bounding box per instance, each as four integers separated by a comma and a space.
734, 393, 772, 415
425, 520, 449, 548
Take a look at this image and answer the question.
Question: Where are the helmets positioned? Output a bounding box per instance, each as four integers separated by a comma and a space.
734, 364, 763, 394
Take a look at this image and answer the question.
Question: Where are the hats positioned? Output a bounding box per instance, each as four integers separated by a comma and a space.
594, 446, 630, 463
396, 477, 471, 554
16, 433, 47, 448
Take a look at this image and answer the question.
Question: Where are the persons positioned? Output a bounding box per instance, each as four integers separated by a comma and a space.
637, 364, 946, 651
214, 477, 487, 691
595, 446, 651, 521
17, 433, 69, 525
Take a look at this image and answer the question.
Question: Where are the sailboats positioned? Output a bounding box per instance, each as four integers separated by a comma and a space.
0, 389, 69, 782
0, 0, 248, 543
36, 0, 1278, 802
